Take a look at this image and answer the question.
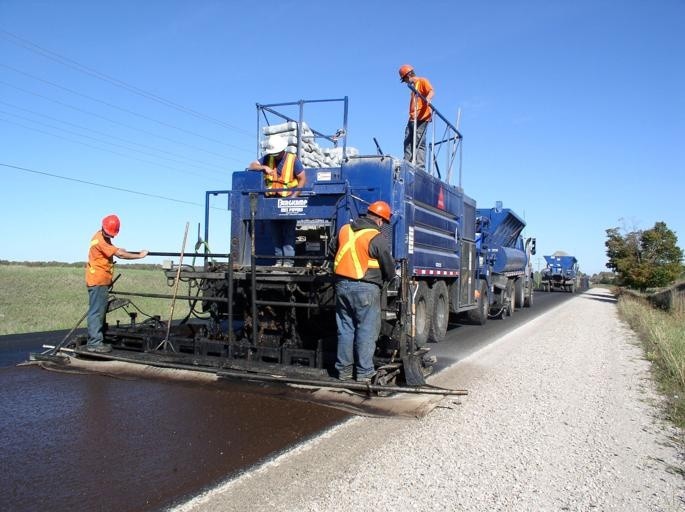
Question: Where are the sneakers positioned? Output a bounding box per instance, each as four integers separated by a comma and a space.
271, 263, 280, 274
357, 371, 377, 381
282, 262, 293, 274
339, 366, 356, 381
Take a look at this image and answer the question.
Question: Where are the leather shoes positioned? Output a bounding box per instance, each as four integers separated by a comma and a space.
87, 343, 112, 352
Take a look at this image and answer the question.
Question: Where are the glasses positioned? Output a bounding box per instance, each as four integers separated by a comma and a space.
268, 154, 280, 157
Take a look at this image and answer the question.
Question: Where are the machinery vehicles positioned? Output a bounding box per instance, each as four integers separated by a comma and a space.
16, 86, 536, 421
541, 255, 578, 294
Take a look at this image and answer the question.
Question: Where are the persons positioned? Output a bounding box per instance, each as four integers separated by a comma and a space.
328, 200, 397, 381
399, 64, 435, 170
250, 135, 306, 267
84, 215, 148, 354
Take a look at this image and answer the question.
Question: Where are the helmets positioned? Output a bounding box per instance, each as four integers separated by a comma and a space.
266, 134, 288, 154
367, 201, 390, 223
102, 215, 120, 238
399, 64, 414, 80
